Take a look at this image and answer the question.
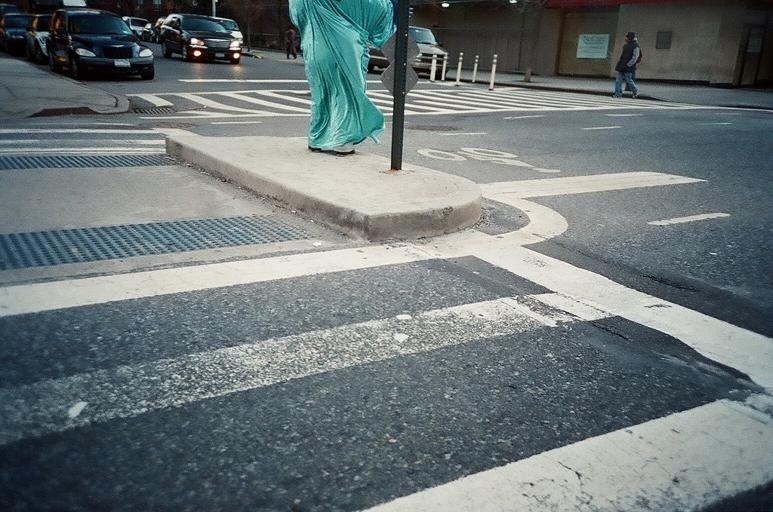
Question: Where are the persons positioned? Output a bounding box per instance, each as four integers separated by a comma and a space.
285, 26, 297, 59
612, 31, 642, 99
289, 0, 397, 155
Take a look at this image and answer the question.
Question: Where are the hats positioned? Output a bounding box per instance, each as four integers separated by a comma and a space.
625, 32, 638, 40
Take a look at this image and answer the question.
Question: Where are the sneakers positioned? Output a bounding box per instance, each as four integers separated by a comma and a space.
322, 144, 354, 152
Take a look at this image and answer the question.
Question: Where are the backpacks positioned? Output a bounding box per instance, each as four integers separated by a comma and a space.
636, 49, 642, 63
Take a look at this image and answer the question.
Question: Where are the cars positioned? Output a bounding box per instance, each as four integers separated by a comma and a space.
158, 9, 242, 65
48, 6, 155, 80
181, 12, 244, 53
121, 15, 166, 42
367, 25, 451, 81
0, 3, 54, 64
